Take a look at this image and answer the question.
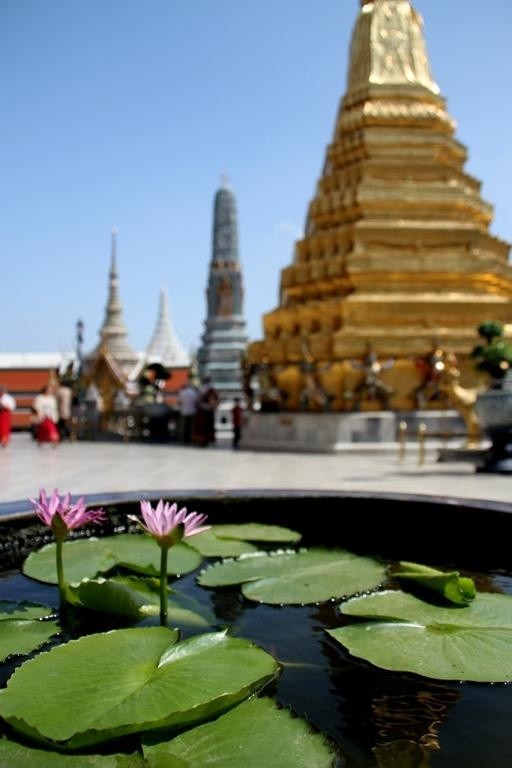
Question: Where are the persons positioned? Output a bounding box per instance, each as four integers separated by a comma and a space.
192, 378, 220, 448
31, 384, 61, 449
232, 398, 243, 449
54, 380, 72, 442
172, 377, 200, 446
1, 385, 18, 447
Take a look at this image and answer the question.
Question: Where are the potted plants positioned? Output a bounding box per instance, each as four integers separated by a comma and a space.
469, 321, 512, 428
